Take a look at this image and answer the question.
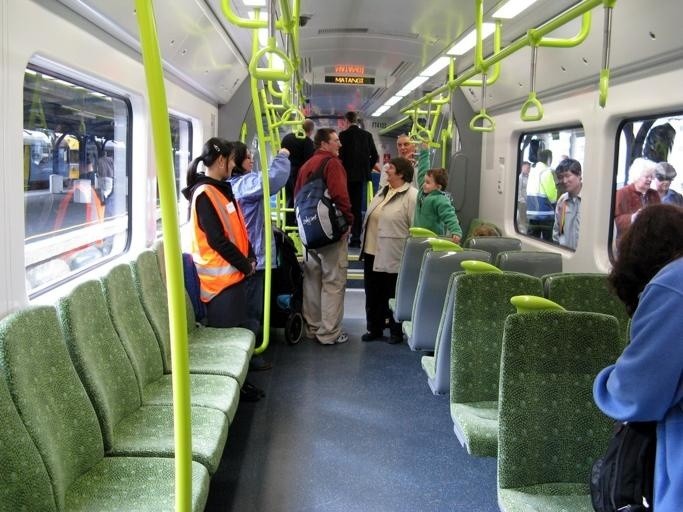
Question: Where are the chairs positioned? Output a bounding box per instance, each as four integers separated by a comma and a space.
450, 272, 632, 511
1, 243, 254, 512
388, 222, 562, 397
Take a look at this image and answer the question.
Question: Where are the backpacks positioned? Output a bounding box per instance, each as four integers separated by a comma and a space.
293, 156, 350, 250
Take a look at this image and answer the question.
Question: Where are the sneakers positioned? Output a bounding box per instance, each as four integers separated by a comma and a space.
361, 329, 384, 342
386, 334, 404, 345
249, 356, 273, 371
326, 332, 350, 344
239, 380, 266, 404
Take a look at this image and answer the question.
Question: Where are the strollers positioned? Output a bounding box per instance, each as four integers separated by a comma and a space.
267, 224, 305, 347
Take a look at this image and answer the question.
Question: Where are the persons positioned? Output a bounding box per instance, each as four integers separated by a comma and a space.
95, 150, 113, 206
52, 138, 114, 270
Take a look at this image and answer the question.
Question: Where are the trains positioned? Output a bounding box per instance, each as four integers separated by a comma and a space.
21, 127, 112, 191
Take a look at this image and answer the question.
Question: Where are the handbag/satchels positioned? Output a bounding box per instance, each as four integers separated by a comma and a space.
588, 420, 658, 512
